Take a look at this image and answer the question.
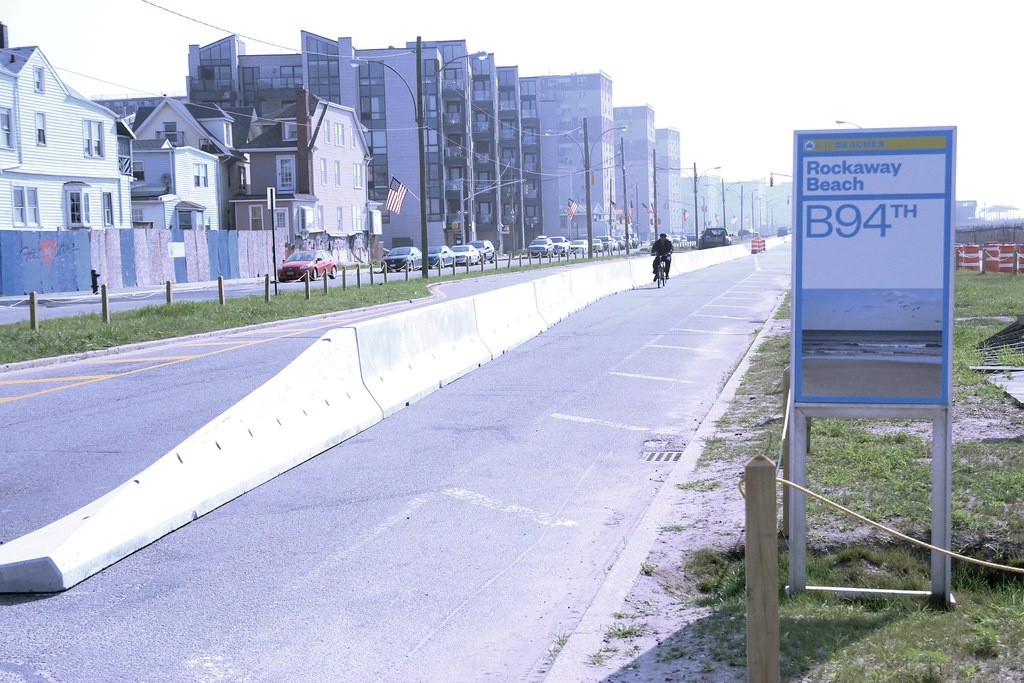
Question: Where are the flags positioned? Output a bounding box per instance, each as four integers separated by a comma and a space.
567, 198, 578, 221
683, 209, 690, 221
385, 176, 408, 214
610, 201, 652, 218
715, 214, 749, 224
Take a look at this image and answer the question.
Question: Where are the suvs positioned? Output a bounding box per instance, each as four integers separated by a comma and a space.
527, 236, 554, 259
468, 240, 494, 264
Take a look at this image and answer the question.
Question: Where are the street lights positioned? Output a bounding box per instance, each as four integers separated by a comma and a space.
702, 181, 779, 242
670, 166, 722, 247
544, 125, 628, 258
348, 52, 490, 281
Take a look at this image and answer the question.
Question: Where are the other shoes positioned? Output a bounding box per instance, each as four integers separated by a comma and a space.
653, 275, 657, 282
666, 275, 669, 279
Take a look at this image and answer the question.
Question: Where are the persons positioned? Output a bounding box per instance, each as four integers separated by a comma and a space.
651, 233, 674, 282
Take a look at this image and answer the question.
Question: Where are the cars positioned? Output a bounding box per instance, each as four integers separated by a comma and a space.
428, 246, 457, 268
738, 230, 752, 240
450, 245, 480, 266
570, 240, 588, 254
277, 250, 338, 283
776, 227, 789, 238
592, 239, 604, 252
550, 236, 571, 257
595, 236, 612, 251
609, 233, 639, 251
381, 247, 422, 272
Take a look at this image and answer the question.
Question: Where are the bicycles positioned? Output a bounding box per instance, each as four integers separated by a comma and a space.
653, 253, 670, 288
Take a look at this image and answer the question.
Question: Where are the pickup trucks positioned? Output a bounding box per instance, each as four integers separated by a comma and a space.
700, 227, 733, 248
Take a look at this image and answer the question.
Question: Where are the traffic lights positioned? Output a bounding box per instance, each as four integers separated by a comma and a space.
770, 177, 773, 187
